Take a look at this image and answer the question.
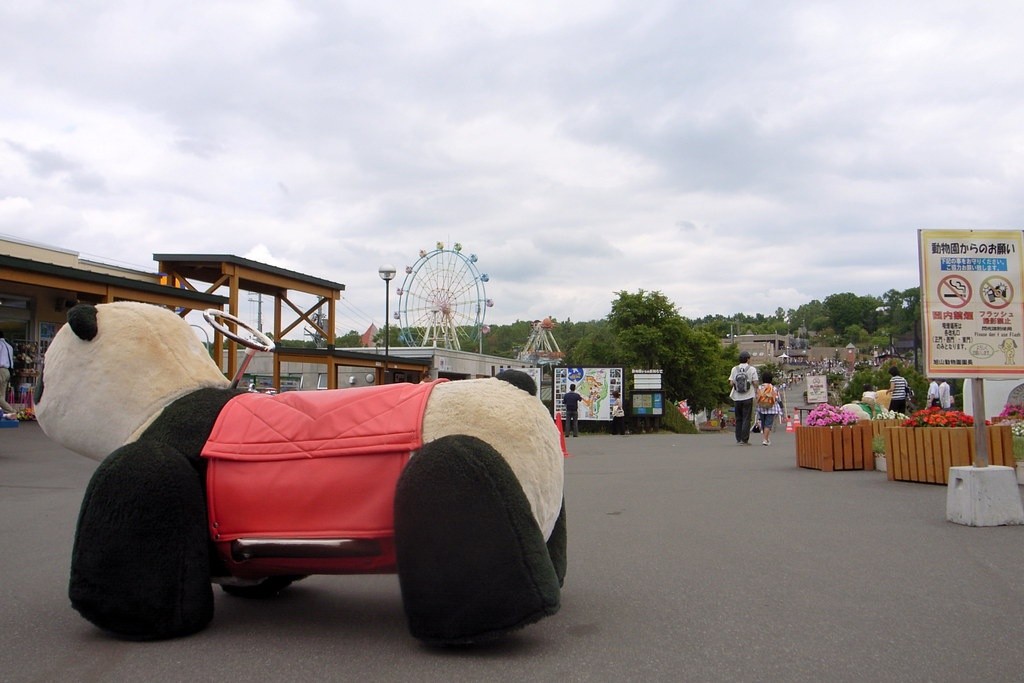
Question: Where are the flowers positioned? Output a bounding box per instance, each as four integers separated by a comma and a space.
805, 402, 859, 427
991, 403, 1024, 436
901, 406, 990, 427
871, 408, 911, 421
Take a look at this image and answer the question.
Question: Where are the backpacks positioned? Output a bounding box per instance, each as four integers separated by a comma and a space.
733, 364, 753, 393
757, 385, 776, 409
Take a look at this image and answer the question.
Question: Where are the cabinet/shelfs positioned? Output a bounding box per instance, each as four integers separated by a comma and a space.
12, 340, 41, 387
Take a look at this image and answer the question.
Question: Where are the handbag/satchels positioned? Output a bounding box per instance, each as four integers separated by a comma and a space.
932, 399, 942, 408
9, 367, 17, 387
750, 413, 761, 433
616, 407, 624, 417
905, 379, 912, 401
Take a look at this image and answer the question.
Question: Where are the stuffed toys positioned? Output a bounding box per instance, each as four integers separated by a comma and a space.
30, 299, 565, 643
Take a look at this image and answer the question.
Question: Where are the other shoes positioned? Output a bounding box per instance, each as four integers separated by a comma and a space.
737, 441, 751, 446
762, 438, 771, 446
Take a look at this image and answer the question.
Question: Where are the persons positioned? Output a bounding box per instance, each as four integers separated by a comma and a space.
925, 378, 950, 411
757, 372, 782, 447
0, 337, 18, 412
564, 384, 583, 438
711, 408, 728, 429
729, 351, 759, 447
886, 366, 908, 415
611, 391, 621, 436
779, 348, 877, 391
419, 371, 431, 383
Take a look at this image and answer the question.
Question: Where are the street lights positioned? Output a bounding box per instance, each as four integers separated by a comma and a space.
377, 263, 397, 384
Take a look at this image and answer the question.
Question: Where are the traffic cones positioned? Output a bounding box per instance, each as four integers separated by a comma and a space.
556, 412, 569, 456
785, 416, 793, 432
792, 408, 801, 431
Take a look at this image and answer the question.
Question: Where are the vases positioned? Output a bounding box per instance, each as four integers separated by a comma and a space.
883, 425, 1016, 484
859, 419, 905, 439
795, 423, 873, 471
